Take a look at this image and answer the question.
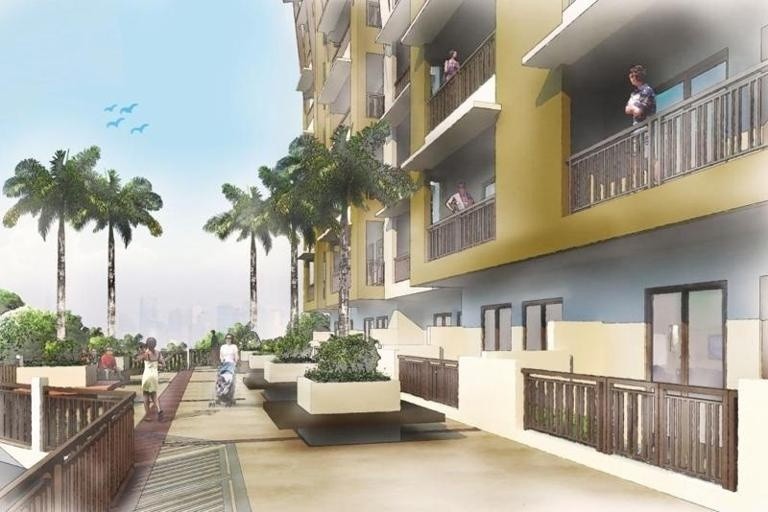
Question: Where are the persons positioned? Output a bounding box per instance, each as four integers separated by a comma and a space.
623, 63, 669, 187
442, 48, 460, 82
102, 346, 126, 382
218, 332, 241, 366
216, 374, 230, 397
131, 335, 168, 424
209, 329, 219, 370
444, 180, 476, 216
